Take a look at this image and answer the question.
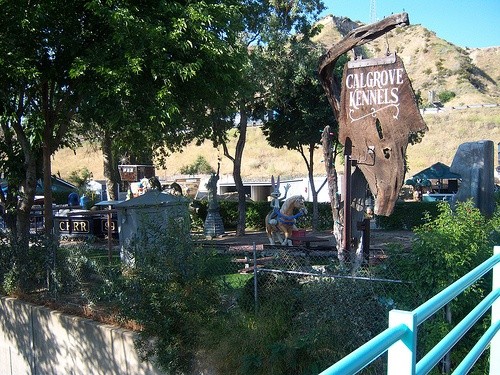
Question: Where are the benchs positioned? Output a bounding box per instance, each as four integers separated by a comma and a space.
194, 241, 248, 253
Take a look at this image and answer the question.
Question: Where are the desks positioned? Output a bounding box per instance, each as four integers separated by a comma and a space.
287, 235, 330, 249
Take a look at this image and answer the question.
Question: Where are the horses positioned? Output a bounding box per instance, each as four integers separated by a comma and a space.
265, 195, 308, 245
